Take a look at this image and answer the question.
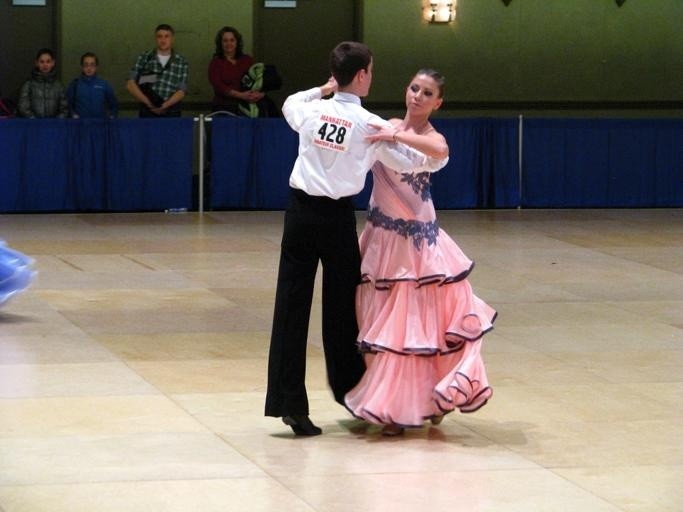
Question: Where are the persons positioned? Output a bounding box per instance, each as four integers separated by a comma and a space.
67, 52, 118, 120
343, 66, 499, 437
17, 47, 69, 118
208, 26, 268, 116
264, 40, 450, 437
125, 24, 189, 118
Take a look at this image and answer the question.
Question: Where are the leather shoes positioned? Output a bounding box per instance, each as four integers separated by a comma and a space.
283, 415, 321, 436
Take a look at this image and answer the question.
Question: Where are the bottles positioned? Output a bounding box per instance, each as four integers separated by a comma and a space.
165, 208, 187, 214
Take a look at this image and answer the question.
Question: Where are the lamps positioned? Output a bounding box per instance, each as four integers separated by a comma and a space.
422, 1, 459, 25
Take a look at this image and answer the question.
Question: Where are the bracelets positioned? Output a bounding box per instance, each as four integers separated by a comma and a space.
393, 129, 400, 145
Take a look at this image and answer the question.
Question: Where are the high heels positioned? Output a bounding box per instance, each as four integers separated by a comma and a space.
383, 424, 405, 435
431, 415, 444, 424
